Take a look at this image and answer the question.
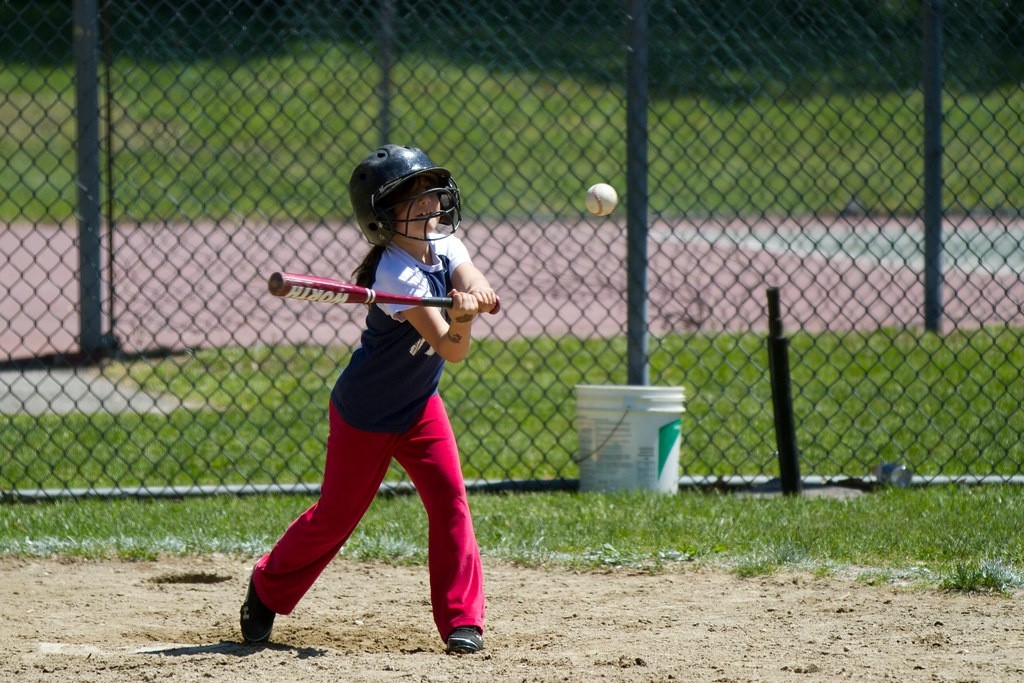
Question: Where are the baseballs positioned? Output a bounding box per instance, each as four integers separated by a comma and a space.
583, 183, 622, 217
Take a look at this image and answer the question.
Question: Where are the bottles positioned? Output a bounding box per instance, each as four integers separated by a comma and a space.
871, 462, 912, 490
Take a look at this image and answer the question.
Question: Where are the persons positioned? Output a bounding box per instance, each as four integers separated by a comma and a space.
237, 144, 498, 657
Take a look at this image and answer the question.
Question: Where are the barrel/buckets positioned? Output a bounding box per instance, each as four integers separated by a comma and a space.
570, 384, 687, 497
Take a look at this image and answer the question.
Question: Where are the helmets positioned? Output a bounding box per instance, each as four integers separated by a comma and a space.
348, 145, 450, 244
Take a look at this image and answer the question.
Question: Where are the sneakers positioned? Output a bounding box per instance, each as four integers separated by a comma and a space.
240, 563, 275, 645
448, 625, 484, 654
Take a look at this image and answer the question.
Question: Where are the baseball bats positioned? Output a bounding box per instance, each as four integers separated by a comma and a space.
268, 269, 501, 317
